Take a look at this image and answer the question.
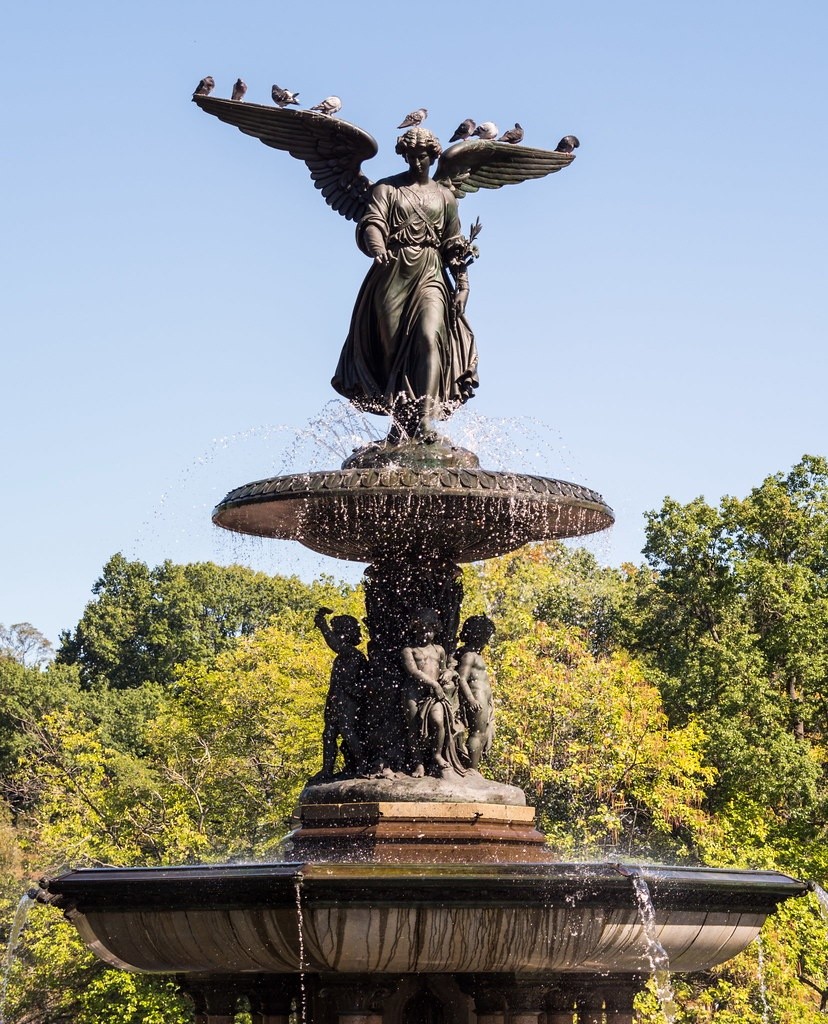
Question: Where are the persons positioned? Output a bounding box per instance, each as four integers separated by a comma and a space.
402, 609, 453, 778
307, 607, 370, 785
330, 127, 480, 442
456, 611, 495, 777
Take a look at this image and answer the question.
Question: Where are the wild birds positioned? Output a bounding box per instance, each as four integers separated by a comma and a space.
190, 75, 216, 103
310, 96, 343, 117
553, 135, 580, 154
449, 118, 524, 144
231, 77, 247, 101
397, 107, 429, 129
272, 84, 301, 108
